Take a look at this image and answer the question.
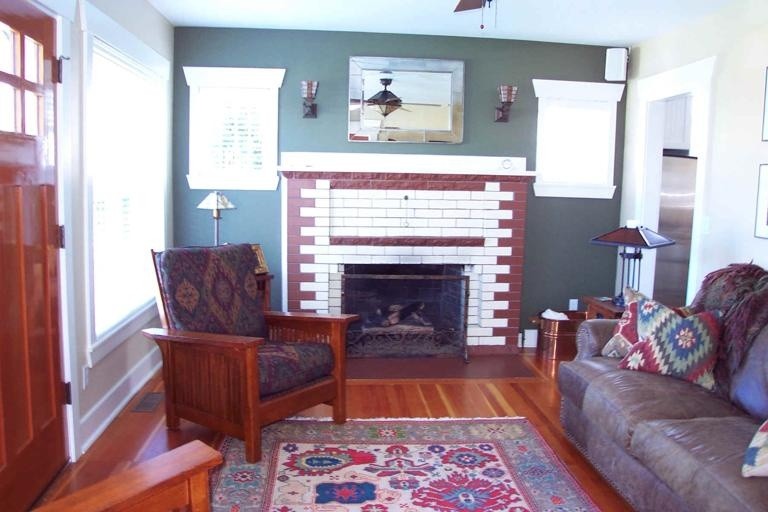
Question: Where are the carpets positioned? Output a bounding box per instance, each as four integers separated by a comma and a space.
209, 416, 604, 512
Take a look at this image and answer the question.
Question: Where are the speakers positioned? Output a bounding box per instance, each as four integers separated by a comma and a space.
604, 48, 628, 82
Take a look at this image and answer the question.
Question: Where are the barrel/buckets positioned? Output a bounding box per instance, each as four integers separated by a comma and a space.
528, 311, 585, 360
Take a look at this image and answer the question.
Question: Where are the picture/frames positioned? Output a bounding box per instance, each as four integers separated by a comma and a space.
753, 163, 768, 240
347, 56, 465, 143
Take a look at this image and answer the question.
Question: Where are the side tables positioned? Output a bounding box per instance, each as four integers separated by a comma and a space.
581, 294, 623, 319
256, 273, 274, 311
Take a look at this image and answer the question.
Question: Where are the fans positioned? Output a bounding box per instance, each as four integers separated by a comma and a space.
350, 79, 441, 112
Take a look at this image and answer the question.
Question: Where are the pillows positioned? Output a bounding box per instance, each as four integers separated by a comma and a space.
600, 285, 698, 358
742, 419, 767, 477
619, 294, 724, 395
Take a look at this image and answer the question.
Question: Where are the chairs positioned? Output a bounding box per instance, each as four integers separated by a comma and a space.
139, 245, 360, 463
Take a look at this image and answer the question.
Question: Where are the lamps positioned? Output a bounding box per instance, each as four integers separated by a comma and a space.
589, 220, 676, 305
367, 104, 400, 117
196, 191, 237, 247
494, 84, 519, 121
299, 80, 320, 118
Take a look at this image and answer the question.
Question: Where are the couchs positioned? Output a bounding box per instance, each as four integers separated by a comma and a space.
558, 261, 768, 512
